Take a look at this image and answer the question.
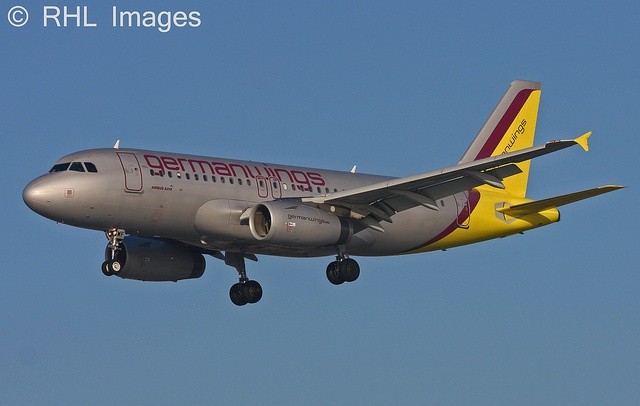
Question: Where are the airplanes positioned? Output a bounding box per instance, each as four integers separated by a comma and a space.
23, 79, 625, 306
